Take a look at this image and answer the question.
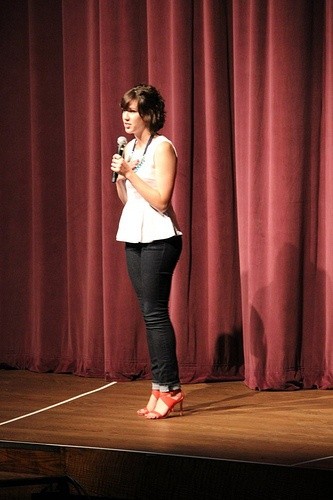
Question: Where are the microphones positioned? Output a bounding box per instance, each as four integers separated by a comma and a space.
112, 137, 127, 183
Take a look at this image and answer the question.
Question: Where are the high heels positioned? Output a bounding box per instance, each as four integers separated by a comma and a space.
136, 389, 185, 419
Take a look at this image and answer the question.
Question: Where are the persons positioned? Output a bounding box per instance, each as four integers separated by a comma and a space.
110, 84, 185, 420
